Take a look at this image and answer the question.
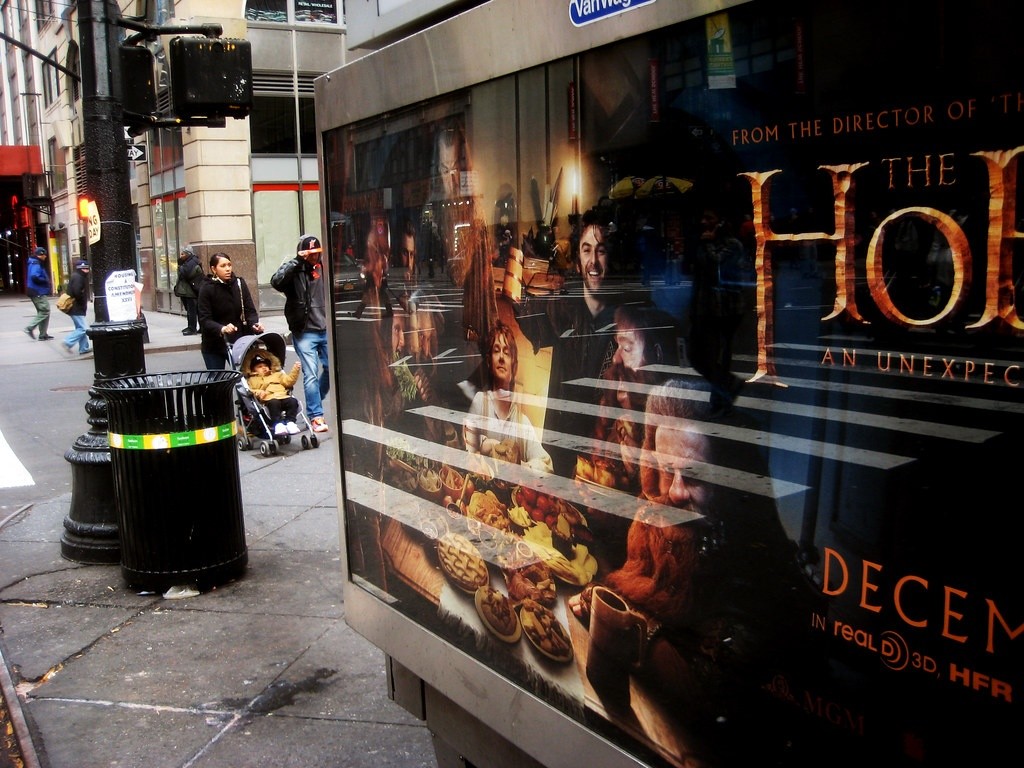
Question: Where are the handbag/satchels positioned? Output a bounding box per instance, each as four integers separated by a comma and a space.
240, 320, 254, 335
56, 293, 74, 314
190, 274, 207, 294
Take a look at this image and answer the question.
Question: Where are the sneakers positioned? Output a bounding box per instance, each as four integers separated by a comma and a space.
311, 417, 328, 432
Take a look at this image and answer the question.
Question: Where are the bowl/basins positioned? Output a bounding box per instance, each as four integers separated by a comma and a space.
409, 473, 418, 494
446, 503, 462, 527
419, 521, 438, 540
417, 470, 442, 500
507, 506, 532, 534
439, 467, 464, 503
477, 531, 497, 557
515, 542, 532, 562
428, 460, 437, 470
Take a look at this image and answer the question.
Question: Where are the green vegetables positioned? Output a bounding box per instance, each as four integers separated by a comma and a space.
386, 437, 414, 464
393, 351, 417, 401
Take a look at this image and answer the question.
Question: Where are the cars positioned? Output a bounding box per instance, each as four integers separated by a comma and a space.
334, 262, 365, 301
447, 244, 564, 296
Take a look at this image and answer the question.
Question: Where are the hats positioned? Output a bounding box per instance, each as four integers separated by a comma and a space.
75, 260, 90, 268
250, 354, 271, 369
302, 237, 323, 254
34, 247, 47, 255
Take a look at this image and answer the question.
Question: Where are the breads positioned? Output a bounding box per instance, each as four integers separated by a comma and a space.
481, 589, 517, 636
501, 552, 557, 608
439, 534, 488, 588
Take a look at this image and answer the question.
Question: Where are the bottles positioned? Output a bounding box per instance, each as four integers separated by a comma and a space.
493, 445, 508, 490
471, 464, 510, 506
535, 226, 555, 259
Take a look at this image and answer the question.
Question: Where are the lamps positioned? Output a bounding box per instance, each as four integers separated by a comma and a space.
49, 221, 65, 232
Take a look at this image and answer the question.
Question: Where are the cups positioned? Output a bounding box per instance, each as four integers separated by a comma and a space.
463, 419, 479, 453
503, 247, 523, 305
586, 586, 647, 688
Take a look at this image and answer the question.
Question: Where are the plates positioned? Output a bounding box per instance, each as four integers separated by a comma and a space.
438, 532, 489, 594
576, 474, 635, 502
475, 586, 521, 643
520, 605, 574, 662
555, 573, 592, 585
438, 515, 450, 540
550, 583, 556, 592
512, 485, 587, 539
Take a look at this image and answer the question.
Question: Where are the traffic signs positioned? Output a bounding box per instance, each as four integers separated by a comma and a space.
127, 144, 147, 162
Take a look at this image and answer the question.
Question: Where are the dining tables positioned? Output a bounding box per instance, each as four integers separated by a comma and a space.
377, 485, 713, 768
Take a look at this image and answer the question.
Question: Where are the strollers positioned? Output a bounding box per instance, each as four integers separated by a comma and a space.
222, 326, 319, 457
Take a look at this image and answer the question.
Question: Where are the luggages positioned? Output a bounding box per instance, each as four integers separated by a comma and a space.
387, 283, 419, 315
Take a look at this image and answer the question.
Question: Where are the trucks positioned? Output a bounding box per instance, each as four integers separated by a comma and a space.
313, 1, 1024, 768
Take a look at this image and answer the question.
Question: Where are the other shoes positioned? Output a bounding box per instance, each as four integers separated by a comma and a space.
38, 335, 54, 341
349, 312, 360, 319
197, 329, 202, 334
79, 348, 93, 356
182, 327, 197, 335
274, 423, 289, 436
23, 326, 36, 340
286, 421, 300, 435
382, 312, 392, 318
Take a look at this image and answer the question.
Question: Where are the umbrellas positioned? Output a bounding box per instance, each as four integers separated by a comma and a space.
608, 176, 646, 199
634, 175, 694, 198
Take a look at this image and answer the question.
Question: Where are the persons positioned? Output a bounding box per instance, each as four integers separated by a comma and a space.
246, 355, 300, 435
173, 246, 204, 335
59, 260, 93, 354
198, 252, 264, 370
25, 247, 55, 340
346, 128, 830, 767
271, 234, 332, 431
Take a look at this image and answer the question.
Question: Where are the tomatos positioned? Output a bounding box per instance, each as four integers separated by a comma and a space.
516, 482, 560, 527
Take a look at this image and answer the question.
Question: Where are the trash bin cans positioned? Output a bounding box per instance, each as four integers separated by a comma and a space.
92, 369, 249, 593
